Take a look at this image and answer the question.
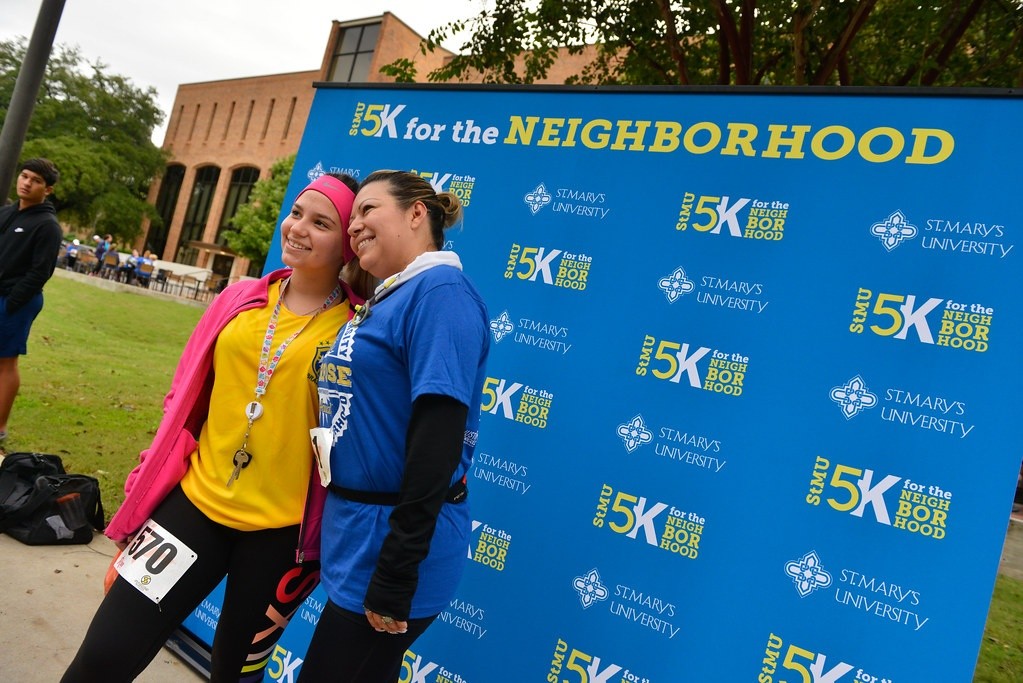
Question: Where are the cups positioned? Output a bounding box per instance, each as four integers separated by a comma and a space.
56, 493, 87, 530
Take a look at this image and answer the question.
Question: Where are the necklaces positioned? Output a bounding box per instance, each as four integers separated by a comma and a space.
300, 307, 320, 316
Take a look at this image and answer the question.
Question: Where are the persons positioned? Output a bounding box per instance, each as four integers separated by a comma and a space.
64, 239, 81, 272
297, 170, 490, 683
0, 159, 63, 454
89, 234, 158, 287
60, 172, 378, 683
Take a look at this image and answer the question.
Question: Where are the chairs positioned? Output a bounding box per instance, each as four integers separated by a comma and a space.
58, 248, 218, 303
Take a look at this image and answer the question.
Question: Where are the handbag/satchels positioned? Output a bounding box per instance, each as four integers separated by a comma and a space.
0, 453, 104, 546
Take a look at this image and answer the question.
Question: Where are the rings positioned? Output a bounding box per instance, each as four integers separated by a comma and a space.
382, 616, 394, 624
362, 605, 372, 613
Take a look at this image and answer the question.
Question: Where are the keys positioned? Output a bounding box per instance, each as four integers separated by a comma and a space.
226, 450, 253, 488
